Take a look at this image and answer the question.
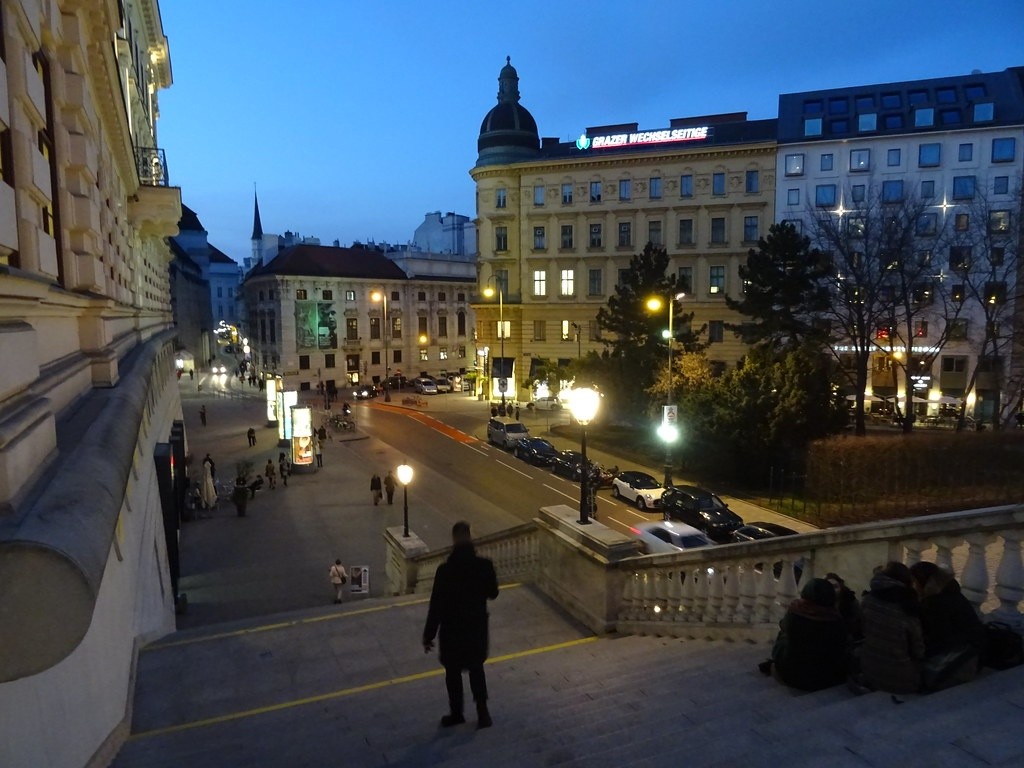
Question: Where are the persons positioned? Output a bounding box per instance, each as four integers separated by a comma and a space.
758, 563, 987, 696
248, 427, 255, 447
317, 381, 351, 414
248, 374, 263, 391
422, 522, 500, 729
384, 471, 397, 504
199, 405, 207, 427
265, 459, 275, 489
370, 473, 381, 506
329, 559, 348, 604
208, 359, 212, 367
229, 482, 248, 516
192, 481, 201, 501
280, 459, 291, 486
491, 403, 513, 417
203, 454, 215, 478
249, 474, 264, 499
189, 370, 193, 380
314, 426, 327, 468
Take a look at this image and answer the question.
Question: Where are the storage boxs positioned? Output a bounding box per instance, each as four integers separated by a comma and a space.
349, 565, 369, 594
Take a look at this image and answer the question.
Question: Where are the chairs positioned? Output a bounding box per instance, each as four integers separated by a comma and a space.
254, 484, 265, 498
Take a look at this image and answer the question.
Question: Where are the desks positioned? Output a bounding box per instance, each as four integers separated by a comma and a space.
871, 413, 879, 415
873, 414, 884, 418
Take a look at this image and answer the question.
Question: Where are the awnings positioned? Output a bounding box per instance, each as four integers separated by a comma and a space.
491, 357, 571, 378
844, 369, 998, 390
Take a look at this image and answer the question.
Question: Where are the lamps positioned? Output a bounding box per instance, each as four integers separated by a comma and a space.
127, 194, 139, 204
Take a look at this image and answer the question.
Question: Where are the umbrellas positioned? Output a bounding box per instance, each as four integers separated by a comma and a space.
201, 461, 217, 512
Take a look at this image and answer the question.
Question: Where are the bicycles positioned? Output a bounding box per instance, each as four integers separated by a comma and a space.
329, 409, 356, 432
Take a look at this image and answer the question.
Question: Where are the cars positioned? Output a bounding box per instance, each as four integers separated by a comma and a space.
613, 471, 665, 509
515, 438, 591, 481
353, 385, 380, 399
729, 522, 799, 542
630, 520, 714, 555
415, 376, 469, 395
527, 396, 563, 412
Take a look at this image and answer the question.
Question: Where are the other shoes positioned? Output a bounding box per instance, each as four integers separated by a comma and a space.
441, 714, 466, 728
759, 659, 774, 676
849, 674, 874, 694
477, 716, 493, 728
334, 599, 342, 604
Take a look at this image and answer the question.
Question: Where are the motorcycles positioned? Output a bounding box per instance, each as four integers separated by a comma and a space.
588, 464, 621, 492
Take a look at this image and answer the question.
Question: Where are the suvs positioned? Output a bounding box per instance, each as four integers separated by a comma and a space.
659, 486, 743, 537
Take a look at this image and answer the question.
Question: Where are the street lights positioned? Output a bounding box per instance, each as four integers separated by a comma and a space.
657, 421, 676, 488
485, 274, 504, 405
370, 291, 393, 402
397, 460, 415, 538
567, 382, 599, 525
646, 283, 676, 489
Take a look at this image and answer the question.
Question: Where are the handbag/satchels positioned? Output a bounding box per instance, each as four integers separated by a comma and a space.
266, 471, 268, 476
273, 476, 276, 484
341, 577, 347, 584
976, 622, 1024, 672
379, 493, 382, 498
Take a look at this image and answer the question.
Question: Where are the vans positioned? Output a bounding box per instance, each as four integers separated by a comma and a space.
486, 416, 532, 449
